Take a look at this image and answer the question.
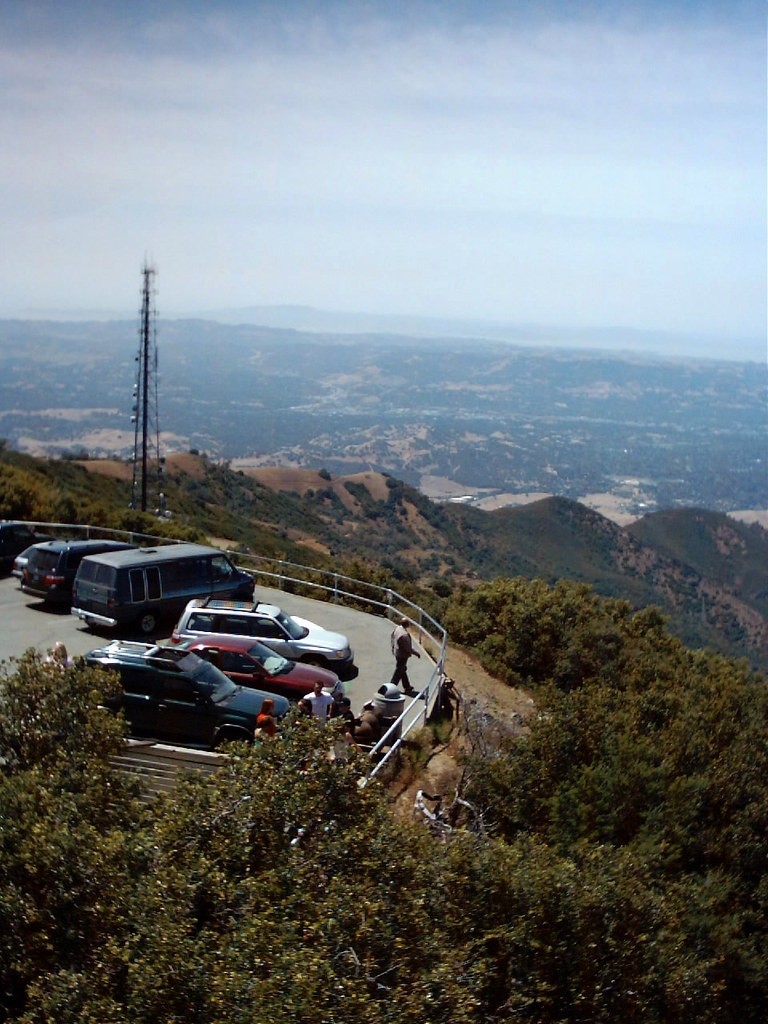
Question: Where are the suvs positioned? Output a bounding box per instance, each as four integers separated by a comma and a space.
172, 596, 354, 673
0, 520, 51, 578
81, 639, 292, 752
21, 539, 140, 612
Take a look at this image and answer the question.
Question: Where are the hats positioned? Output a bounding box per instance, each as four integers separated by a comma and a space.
336, 698, 351, 707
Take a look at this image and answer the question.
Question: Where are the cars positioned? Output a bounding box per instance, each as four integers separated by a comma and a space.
154, 634, 343, 702
11, 546, 31, 580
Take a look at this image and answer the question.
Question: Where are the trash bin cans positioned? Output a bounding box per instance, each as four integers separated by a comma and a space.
372, 683, 407, 747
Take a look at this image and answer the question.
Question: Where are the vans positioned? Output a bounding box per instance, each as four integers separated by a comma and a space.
69, 542, 256, 640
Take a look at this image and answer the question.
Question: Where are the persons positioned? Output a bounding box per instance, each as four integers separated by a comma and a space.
46, 642, 72, 666
389, 617, 421, 692
256, 682, 363, 763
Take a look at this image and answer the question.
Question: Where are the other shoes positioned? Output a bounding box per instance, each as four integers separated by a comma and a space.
405, 687, 413, 691
